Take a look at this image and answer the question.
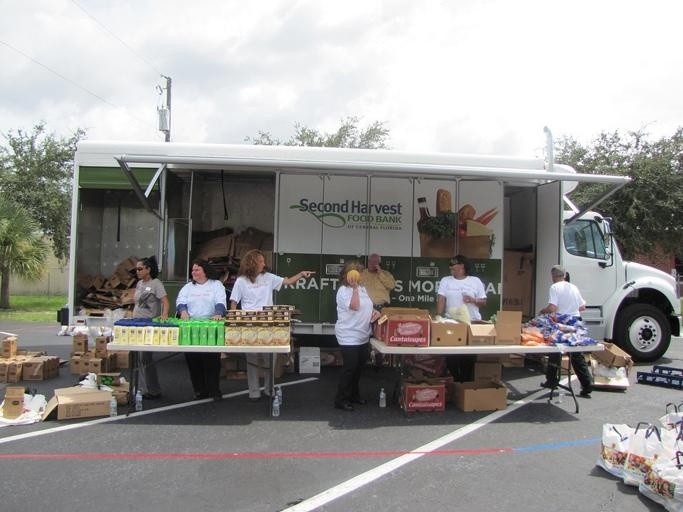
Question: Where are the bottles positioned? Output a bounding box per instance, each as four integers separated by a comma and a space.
272, 396, 280, 417
134, 389, 142, 410
276, 385, 282, 405
378, 387, 386, 408
416, 196, 431, 220
460, 288, 468, 299
108, 395, 119, 418
558, 386, 566, 405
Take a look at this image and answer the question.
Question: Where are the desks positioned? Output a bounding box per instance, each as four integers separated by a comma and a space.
369, 339, 606, 421
105, 340, 291, 419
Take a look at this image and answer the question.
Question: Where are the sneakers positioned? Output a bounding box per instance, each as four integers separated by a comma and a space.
541, 381, 558, 389
580, 389, 591, 395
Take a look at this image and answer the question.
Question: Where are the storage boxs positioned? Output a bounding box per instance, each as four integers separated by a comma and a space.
220, 352, 284, 381
372, 302, 524, 414
500, 248, 535, 317
296, 346, 321, 374
0, 328, 131, 423
192, 224, 272, 272
541, 338, 632, 387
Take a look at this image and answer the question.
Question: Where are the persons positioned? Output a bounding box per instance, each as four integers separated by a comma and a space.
333, 260, 381, 412
229, 248, 316, 403
175, 257, 226, 402
435, 255, 487, 321
538, 265, 592, 396
130, 255, 169, 401
360, 254, 395, 309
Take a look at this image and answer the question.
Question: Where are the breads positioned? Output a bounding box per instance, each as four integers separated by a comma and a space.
436, 188, 452, 216
456, 203, 476, 229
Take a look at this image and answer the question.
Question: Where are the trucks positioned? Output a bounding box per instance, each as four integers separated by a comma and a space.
50, 126, 681, 371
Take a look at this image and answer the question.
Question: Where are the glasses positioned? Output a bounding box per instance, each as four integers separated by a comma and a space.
136, 267, 142, 270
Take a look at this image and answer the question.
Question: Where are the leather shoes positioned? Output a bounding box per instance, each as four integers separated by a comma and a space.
142, 392, 367, 409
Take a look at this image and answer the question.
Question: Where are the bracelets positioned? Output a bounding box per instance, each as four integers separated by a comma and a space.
540, 310, 543, 314
